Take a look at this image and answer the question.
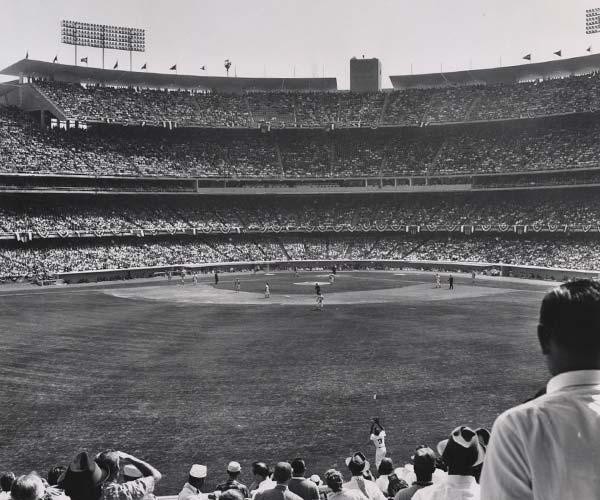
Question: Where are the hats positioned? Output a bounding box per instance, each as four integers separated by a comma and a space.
57, 451, 110, 491
411, 447, 437, 469
228, 460, 241, 473
437, 426, 486, 468
189, 463, 207, 478
346, 452, 370, 472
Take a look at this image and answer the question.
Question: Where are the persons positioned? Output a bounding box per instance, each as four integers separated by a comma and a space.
2, 414, 491, 500
0, 73, 599, 310
476, 278, 600, 500
